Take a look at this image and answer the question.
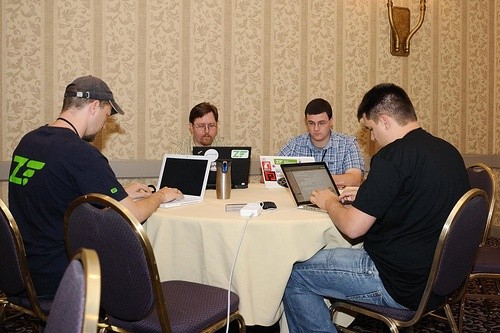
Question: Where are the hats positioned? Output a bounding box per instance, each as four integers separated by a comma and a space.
63, 75, 125, 116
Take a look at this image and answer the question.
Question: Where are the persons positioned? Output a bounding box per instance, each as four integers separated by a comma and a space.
168, 102, 232, 156
281, 83, 471, 333
8, 75, 184, 301
260, 98, 365, 189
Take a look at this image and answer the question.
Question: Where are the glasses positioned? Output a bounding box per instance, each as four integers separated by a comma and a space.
306, 119, 332, 127
193, 122, 217, 129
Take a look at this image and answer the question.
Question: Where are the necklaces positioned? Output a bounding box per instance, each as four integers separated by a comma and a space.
56, 118, 80, 138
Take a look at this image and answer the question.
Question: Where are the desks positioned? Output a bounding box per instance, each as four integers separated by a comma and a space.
140, 182, 365, 333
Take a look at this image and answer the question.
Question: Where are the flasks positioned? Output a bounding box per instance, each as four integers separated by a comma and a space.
214, 158, 232, 199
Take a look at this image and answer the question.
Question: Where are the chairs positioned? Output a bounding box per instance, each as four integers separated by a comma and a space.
0, 164, 500, 333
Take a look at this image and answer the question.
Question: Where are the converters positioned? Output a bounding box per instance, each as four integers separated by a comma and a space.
240, 202, 263, 216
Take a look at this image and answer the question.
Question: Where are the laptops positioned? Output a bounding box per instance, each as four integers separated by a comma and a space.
193, 146, 251, 189
133, 154, 212, 208
260, 156, 316, 188
281, 162, 354, 213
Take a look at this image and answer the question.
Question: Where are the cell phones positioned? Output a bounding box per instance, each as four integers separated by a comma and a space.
260, 202, 277, 209
